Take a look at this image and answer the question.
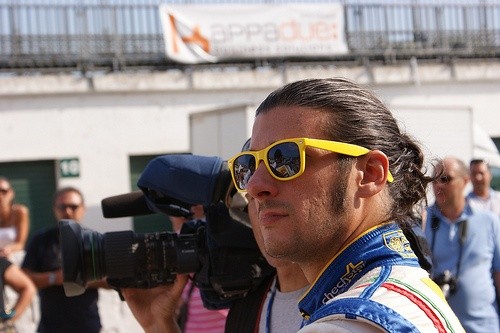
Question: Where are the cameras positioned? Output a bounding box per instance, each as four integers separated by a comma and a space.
433, 270, 456, 297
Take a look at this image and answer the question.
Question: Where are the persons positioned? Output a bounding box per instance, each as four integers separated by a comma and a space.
119, 77, 466, 333
411, 156, 500, 333
19, 187, 114, 333
168, 204, 258, 333
0, 175, 41, 333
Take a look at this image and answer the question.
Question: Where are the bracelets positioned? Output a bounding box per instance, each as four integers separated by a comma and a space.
48, 271, 56, 286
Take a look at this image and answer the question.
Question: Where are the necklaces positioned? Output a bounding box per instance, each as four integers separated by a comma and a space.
266, 278, 305, 333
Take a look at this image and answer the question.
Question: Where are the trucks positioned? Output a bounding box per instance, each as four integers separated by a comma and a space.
186, 102, 500, 202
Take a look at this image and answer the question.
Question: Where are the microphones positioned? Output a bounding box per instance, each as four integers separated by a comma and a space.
101, 189, 155, 218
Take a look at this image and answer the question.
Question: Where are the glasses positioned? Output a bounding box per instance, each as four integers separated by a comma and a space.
0, 187, 11, 194
431, 174, 464, 182
55, 203, 82, 211
228, 137, 393, 192
